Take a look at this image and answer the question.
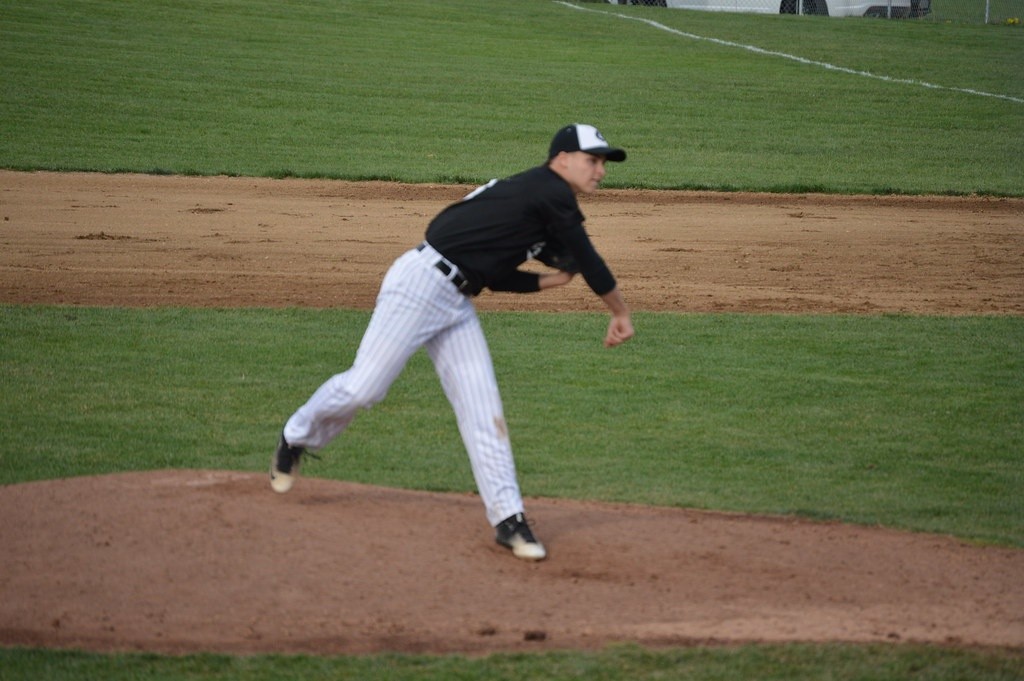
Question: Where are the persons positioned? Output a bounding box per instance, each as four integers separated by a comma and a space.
269, 123, 634, 562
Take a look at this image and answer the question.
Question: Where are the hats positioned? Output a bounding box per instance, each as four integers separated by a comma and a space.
549, 123, 626, 162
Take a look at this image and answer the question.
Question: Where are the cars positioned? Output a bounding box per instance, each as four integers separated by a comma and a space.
608, 0, 935, 18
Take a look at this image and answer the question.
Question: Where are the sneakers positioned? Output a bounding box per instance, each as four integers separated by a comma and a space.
269, 430, 322, 493
494, 513, 547, 560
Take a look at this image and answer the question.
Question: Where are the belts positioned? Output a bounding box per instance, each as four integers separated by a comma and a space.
417, 244, 471, 296
